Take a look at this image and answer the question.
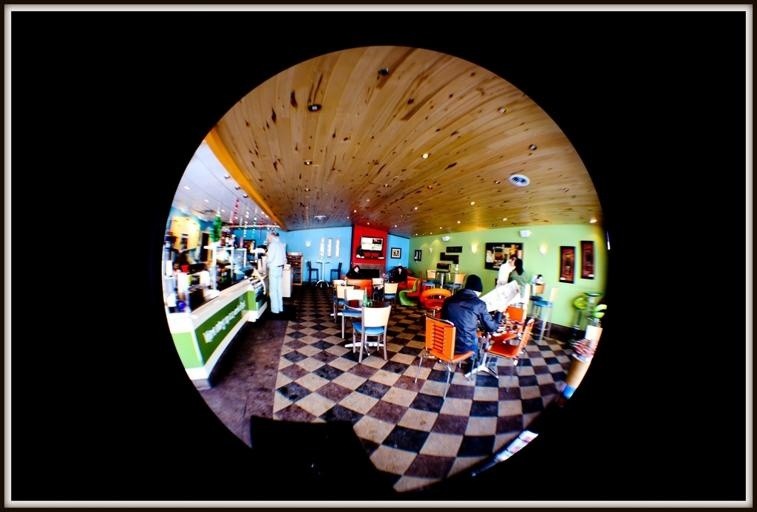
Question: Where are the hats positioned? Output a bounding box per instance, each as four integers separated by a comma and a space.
465, 275, 482, 292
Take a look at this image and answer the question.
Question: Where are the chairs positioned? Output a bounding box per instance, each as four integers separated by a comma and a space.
306, 261, 558, 401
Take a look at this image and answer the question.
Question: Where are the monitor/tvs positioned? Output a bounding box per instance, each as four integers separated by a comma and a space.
360, 236, 384, 252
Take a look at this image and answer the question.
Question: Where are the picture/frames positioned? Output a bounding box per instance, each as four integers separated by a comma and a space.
559, 241, 594, 283
391, 248, 401, 259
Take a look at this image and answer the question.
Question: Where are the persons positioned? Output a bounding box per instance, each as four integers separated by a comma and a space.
507, 258, 531, 339
496, 255, 517, 290
441, 275, 502, 378
265, 231, 285, 314
391, 266, 405, 283
344, 266, 362, 279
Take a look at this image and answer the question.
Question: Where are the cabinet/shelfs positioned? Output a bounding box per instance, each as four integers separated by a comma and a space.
288, 252, 302, 286
